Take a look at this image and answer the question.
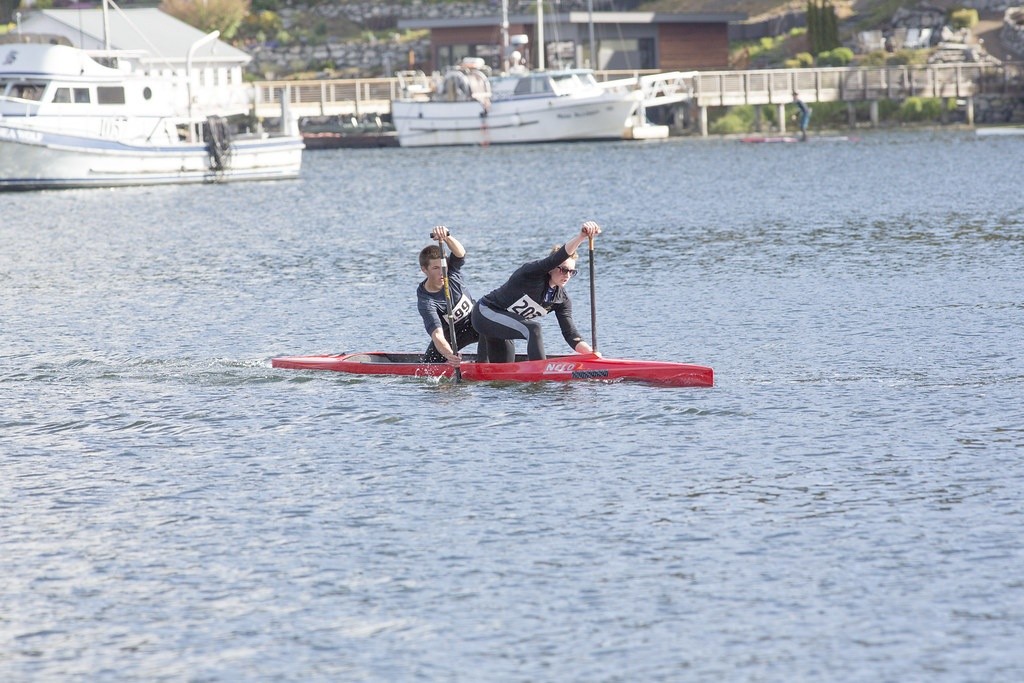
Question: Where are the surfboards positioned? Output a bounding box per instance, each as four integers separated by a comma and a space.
739, 136, 861, 143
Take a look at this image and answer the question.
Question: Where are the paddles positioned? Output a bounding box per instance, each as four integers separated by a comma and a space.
431, 230, 463, 385
581, 225, 602, 355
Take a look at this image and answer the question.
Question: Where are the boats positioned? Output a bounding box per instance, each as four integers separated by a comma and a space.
391, 63, 640, 147
0, 0, 306, 190
271, 349, 716, 387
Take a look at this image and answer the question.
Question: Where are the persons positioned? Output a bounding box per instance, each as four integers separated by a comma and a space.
415, 226, 478, 364
471, 220, 602, 362
789, 90, 812, 142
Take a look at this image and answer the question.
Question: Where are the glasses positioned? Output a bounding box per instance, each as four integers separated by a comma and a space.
557, 266, 577, 277
